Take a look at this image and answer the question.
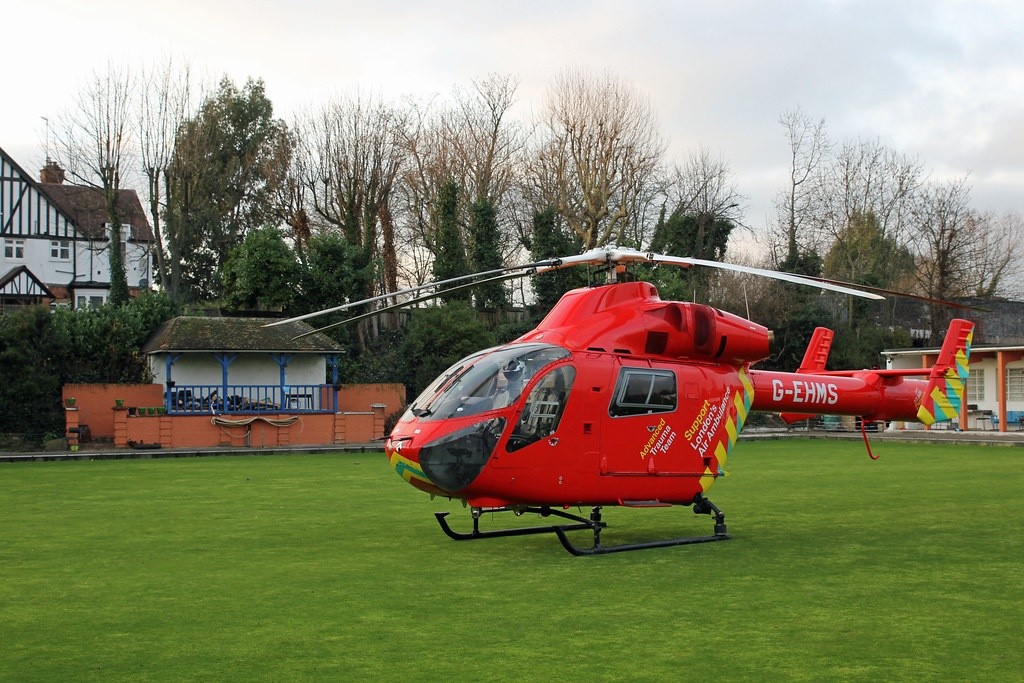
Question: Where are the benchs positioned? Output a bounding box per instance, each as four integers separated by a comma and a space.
994, 411, 1024, 431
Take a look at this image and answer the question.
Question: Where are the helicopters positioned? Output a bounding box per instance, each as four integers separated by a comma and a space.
258, 246, 990, 555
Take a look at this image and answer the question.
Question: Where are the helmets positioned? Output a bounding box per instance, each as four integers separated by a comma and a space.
501, 360, 526, 382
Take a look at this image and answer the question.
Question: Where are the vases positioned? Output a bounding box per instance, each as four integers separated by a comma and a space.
115, 399, 124, 406
148, 407, 156, 414
157, 406, 166, 414
129, 406, 137, 415
165, 381, 176, 387
137, 407, 146, 415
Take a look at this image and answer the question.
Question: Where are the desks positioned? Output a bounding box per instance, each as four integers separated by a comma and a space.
285, 394, 313, 409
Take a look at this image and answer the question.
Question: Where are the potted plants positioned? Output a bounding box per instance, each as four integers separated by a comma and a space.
65, 396, 77, 408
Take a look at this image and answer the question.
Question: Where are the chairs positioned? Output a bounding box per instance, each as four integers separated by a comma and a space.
976, 410, 992, 430
523, 369, 563, 439
179, 390, 195, 404
165, 393, 180, 407
